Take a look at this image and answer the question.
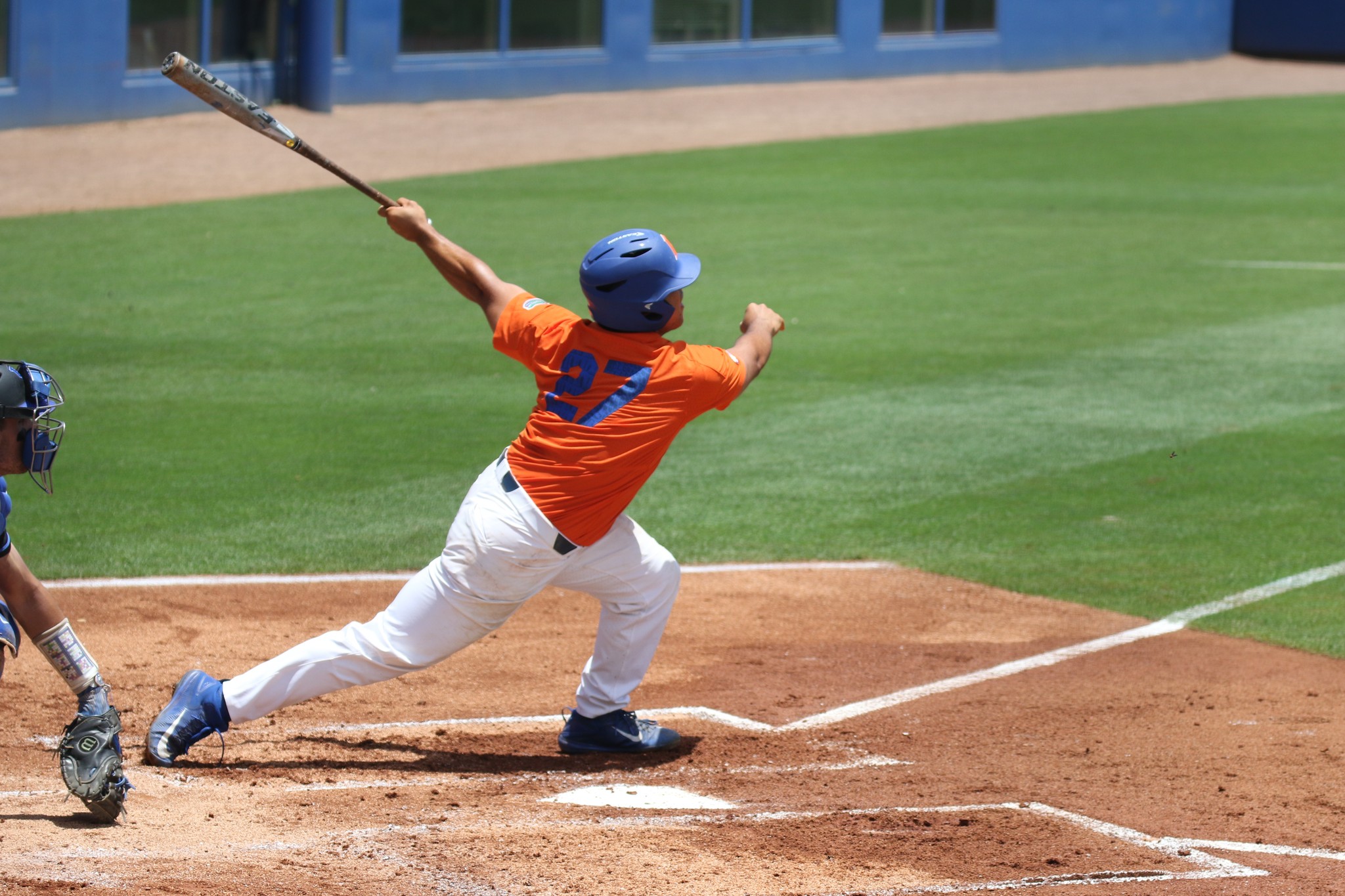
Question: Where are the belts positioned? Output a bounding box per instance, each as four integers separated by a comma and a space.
494, 454, 577, 556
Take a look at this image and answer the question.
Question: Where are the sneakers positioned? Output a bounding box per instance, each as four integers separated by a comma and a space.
146, 670, 231, 766
559, 708, 680, 753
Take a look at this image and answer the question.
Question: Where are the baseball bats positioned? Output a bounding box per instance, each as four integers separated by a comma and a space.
160, 50, 433, 229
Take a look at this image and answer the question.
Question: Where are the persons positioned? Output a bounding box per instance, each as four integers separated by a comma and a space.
145, 197, 787, 766
0, 360, 134, 822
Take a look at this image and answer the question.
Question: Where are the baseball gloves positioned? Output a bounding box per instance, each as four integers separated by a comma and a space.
59, 706, 136, 824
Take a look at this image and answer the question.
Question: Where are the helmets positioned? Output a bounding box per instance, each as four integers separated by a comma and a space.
579, 228, 702, 333
0, 361, 41, 420
